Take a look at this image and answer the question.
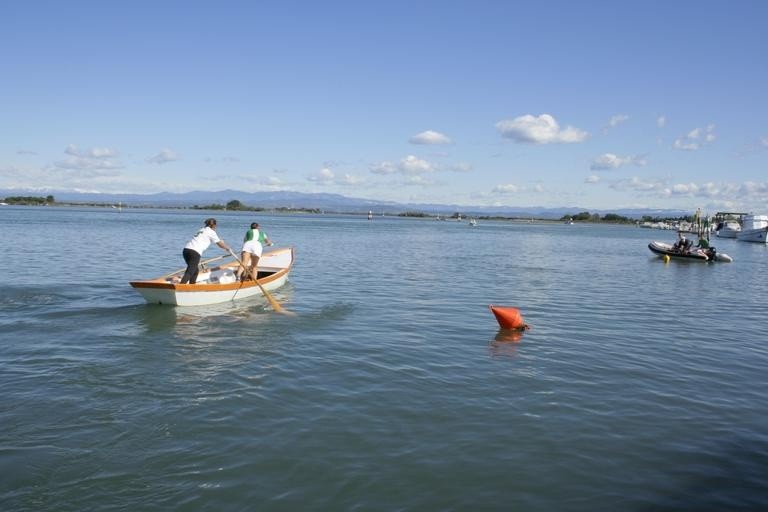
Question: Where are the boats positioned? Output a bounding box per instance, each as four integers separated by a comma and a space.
147, 280, 295, 326
712, 220, 741, 239
126, 245, 297, 306
648, 240, 733, 265
734, 211, 768, 244
638, 220, 716, 235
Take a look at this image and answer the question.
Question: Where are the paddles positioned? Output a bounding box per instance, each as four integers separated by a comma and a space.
228, 249, 294, 317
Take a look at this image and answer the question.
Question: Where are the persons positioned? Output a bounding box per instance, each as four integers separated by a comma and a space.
180, 218, 229, 285
674, 232, 690, 254
694, 234, 710, 261
236, 222, 271, 284
